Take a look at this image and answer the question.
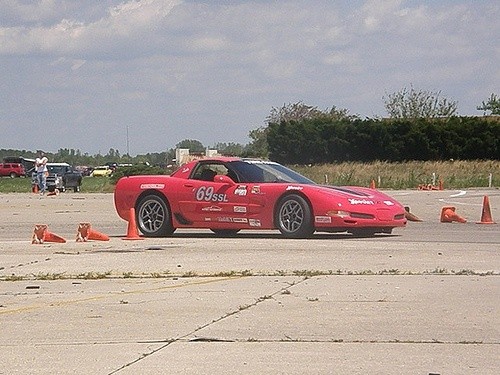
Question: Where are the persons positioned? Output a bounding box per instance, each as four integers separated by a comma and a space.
34, 150, 49, 194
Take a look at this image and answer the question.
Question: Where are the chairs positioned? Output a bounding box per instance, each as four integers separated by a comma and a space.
202, 168, 218, 181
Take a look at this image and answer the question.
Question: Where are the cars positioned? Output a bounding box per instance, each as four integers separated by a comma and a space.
93, 165, 111, 178
75, 166, 90, 176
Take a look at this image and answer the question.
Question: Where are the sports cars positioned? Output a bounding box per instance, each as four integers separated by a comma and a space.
116, 156, 408, 240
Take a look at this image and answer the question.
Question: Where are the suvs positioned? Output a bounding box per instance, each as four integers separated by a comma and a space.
0, 162, 26, 179
32, 163, 82, 193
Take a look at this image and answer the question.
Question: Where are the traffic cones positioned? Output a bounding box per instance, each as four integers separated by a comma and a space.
77, 222, 110, 241
417, 179, 443, 190
441, 207, 466, 223
32, 224, 66, 244
370, 179, 375, 189
122, 208, 144, 241
47, 188, 60, 196
475, 196, 495, 223
405, 206, 423, 222
33, 184, 39, 194
